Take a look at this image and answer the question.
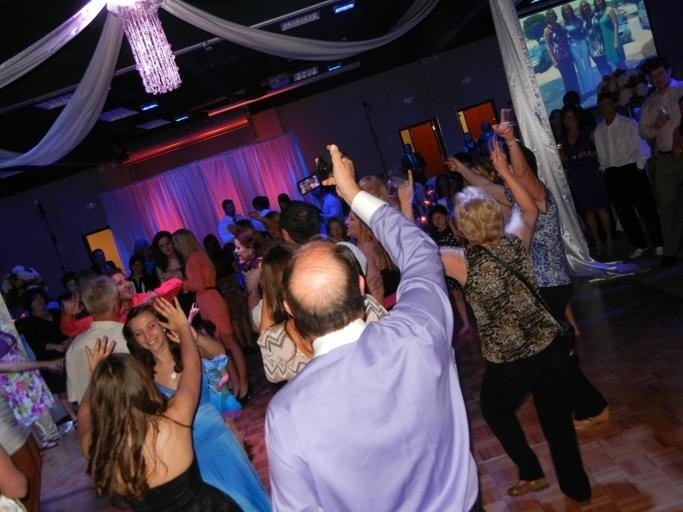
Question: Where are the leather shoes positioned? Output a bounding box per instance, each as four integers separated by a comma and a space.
238, 382, 251, 405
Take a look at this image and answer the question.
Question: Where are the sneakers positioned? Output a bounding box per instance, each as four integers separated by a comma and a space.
507, 477, 547, 496
572, 407, 609, 428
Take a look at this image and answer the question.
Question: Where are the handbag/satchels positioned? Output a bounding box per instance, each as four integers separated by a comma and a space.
552, 319, 576, 345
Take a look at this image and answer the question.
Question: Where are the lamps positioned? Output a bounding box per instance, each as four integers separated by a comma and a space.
107, 0, 185, 98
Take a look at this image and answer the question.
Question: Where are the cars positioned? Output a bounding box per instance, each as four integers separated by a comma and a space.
636, 0, 650, 30
538, 25, 575, 41
617, 12, 631, 44
526, 42, 554, 73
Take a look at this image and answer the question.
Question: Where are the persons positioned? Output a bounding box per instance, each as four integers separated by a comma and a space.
544, 0, 629, 102
0, 193, 403, 512
549, 60, 681, 259
264, 144, 484, 512
355, 107, 610, 503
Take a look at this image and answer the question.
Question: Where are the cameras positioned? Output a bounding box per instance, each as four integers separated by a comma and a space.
297, 148, 333, 195
